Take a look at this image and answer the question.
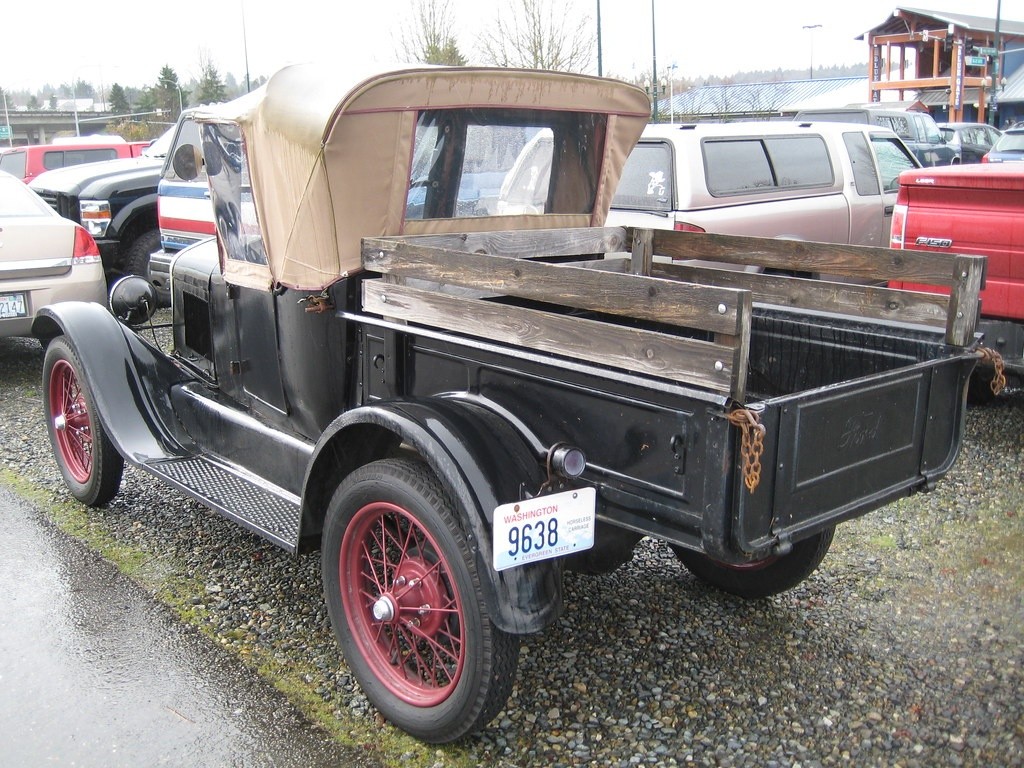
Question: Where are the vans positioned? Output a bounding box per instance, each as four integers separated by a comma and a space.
496, 123, 929, 288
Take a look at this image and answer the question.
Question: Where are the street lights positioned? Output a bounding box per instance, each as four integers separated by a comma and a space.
802, 24, 822, 79
644, 78, 667, 123
982, 72, 1006, 126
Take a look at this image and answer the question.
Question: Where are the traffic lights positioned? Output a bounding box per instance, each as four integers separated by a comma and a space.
945, 32, 954, 52
965, 36, 973, 55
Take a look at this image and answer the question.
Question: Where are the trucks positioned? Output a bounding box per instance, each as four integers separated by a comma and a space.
29, 59, 985, 743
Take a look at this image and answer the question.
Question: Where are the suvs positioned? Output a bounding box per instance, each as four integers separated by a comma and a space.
982, 122, 1024, 164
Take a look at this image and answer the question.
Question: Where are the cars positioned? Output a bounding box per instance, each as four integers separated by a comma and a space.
0, 169, 109, 337
936, 123, 1002, 163
888, 163, 1024, 401
147, 108, 537, 294
27, 124, 179, 306
793, 107, 960, 168
0, 142, 153, 184
